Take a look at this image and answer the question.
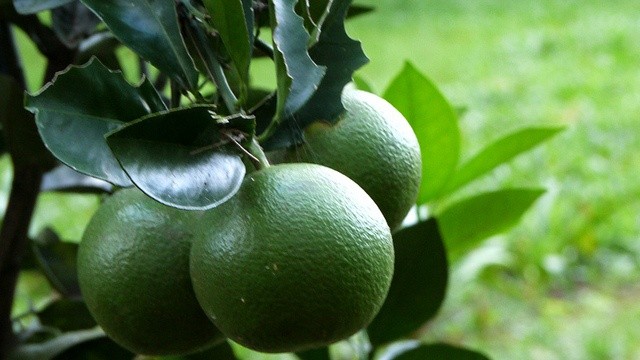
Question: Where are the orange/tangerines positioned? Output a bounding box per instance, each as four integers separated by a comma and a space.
265, 86, 422, 231
189, 162, 396, 352
76, 186, 228, 356
